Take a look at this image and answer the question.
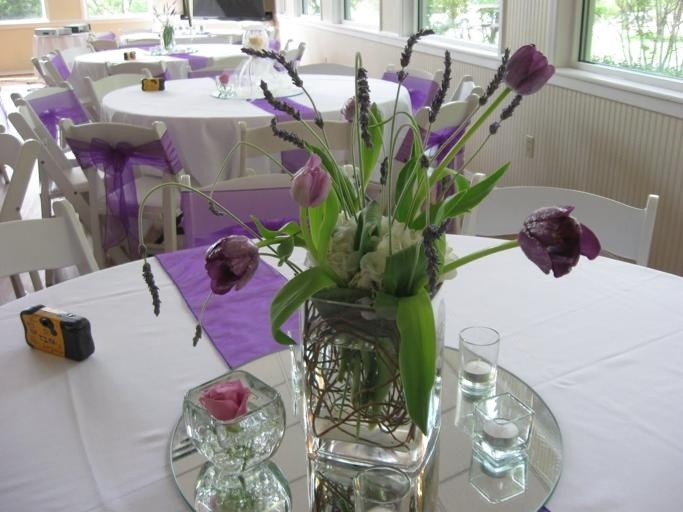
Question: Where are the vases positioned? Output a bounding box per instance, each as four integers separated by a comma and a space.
275, 257, 444, 478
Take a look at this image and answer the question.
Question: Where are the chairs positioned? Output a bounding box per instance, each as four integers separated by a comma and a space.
0, 21, 661, 317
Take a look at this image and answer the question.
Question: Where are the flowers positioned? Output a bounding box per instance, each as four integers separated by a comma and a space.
195, 375, 256, 436
115, 24, 619, 455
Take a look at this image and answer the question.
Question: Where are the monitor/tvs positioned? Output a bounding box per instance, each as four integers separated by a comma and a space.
189, 0, 275, 18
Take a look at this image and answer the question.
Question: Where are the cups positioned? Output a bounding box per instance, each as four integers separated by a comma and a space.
474, 393, 534, 469
455, 397, 488, 438
349, 463, 410, 512
457, 327, 501, 400
285, 329, 310, 393
468, 451, 527, 503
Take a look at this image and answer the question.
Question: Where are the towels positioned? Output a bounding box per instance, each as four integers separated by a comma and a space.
148, 236, 300, 374
